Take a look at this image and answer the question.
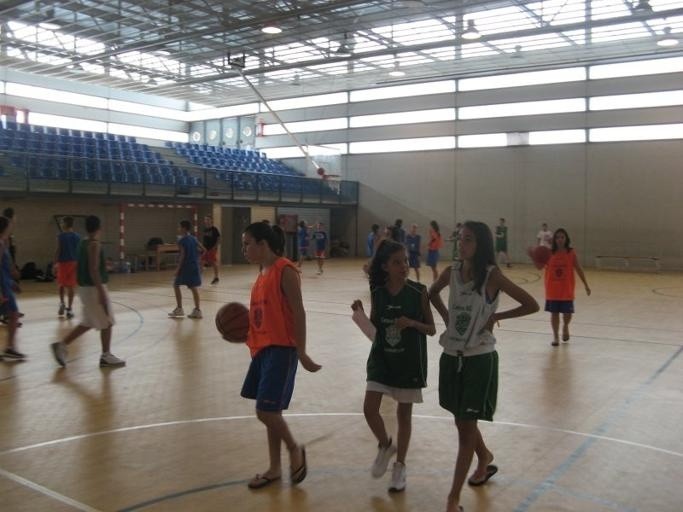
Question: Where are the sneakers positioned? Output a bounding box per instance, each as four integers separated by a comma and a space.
168, 308, 184, 316
67, 308, 73, 317
0, 347, 23, 358
59, 303, 65, 315
372, 436, 396, 478
0, 319, 21, 327
188, 309, 202, 318
5, 312, 23, 317
211, 278, 219, 284
100, 353, 125, 367
389, 462, 406, 491
52, 343, 67, 366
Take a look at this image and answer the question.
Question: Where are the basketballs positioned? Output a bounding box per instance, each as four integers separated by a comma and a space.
533, 245, 550, 263
317, 167, 325, 175
216, 303, 250, 340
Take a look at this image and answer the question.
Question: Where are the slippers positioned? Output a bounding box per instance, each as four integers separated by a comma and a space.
469, 465, 497, 484
249, 473, 281, 489
551, 341, 559, 345
563, 334, 569, 341
291, 445, 306, 483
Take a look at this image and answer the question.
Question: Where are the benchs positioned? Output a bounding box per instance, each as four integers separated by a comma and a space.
595, 254, 660, 273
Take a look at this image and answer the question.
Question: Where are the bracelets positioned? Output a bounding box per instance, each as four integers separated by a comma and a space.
491, 312, 498, 323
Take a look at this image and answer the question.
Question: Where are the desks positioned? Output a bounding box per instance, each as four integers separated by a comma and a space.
143, 241, 203, 271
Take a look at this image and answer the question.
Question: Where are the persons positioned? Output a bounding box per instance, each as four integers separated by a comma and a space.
350, 238, 436, 492
0, 206, 25, 329
199, 215, 221, 285
493, 217, 514, 268
363, 218, 461, 284
304, 221, 330, 275
221, 221, 323, 488
527, 227, 591, 346
56, 215, 81, 318
294, 221, 317, 268
167, 219, 208, 318
0, 214, 26, 360
430, 220, 541, 511
534, 221, 556, 255
50, 214, 126, 368
